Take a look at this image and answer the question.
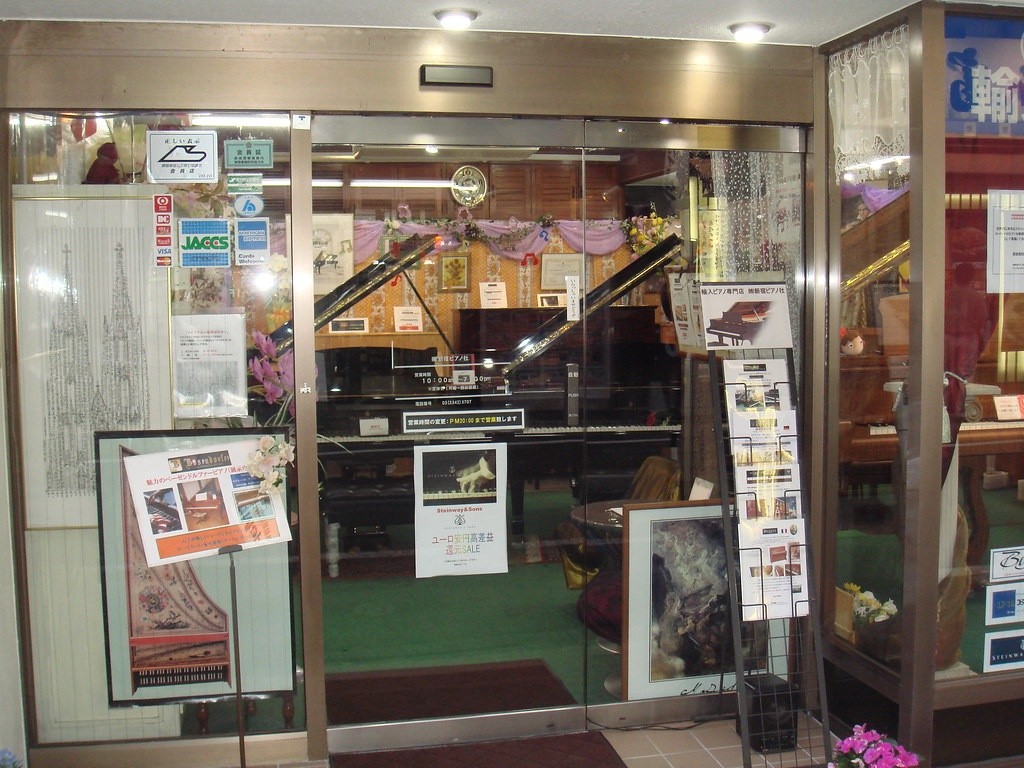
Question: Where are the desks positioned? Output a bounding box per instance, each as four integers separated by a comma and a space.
574, 499, 663, 646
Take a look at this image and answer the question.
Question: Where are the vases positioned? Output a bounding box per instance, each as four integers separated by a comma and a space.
857, 611, 896, 661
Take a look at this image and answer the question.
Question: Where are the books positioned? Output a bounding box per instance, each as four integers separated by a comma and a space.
721, 355, 814, 623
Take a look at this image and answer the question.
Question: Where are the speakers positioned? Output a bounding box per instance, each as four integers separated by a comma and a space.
735, 673, 800, 753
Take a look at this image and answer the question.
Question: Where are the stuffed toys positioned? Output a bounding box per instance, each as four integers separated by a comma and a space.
839, 327, 864, 356
84, 141, 122, 184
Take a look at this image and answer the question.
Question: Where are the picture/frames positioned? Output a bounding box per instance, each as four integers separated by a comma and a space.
540, 252, 583, 291
94, 426, 297, 708
620, 502, 798, 702
438, 252, 472, 293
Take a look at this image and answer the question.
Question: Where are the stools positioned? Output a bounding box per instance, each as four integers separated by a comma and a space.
571, 461, 651, 507
320, 476, 416, 579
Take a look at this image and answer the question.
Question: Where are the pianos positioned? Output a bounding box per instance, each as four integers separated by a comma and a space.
704, 300, 773, 347
456, 302, 663, 386
184, 493, 225, 521
245, 232, 498, 481
493, 233, 685, 538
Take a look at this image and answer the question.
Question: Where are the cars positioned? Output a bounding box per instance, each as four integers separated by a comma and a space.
247, 366, 454, 454
480, 355, 612, 411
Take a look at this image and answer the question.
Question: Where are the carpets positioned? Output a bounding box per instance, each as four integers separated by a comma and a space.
316, 657, 628, 768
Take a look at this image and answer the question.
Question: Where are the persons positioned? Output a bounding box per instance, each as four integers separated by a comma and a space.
455, 452, 497, 495
944, 263, 990, 420
147, 488, 180, 533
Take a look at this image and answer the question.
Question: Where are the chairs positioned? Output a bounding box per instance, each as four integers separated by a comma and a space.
557, 457, 684, 572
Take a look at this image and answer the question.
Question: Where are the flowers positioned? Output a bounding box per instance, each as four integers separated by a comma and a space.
826, 723, 920, 768
845, 582, 899, 625
383, 202, 678, 260
0, 746, 25, 768
248, 329, 297, 492
139, 162, 235, 311
